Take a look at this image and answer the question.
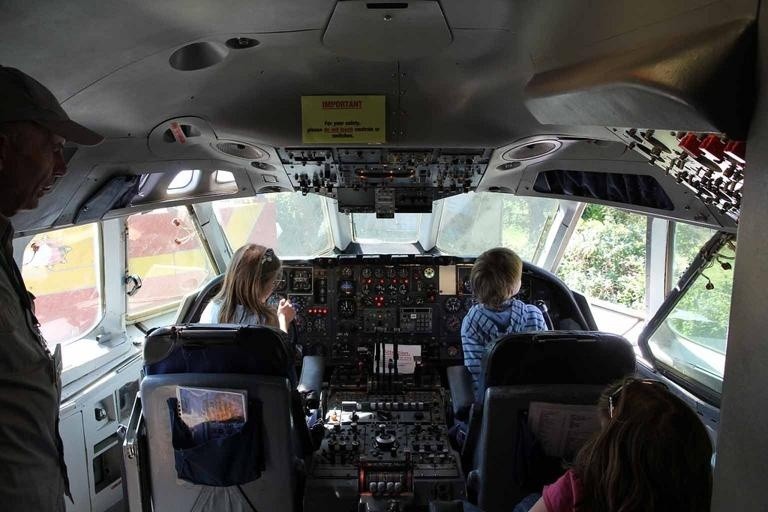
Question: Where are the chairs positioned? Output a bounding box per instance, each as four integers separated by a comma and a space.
139, 324, 326, 511
445, 330, 637, 511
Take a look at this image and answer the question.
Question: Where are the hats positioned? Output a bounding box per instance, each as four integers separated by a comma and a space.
0, 65, 105, 146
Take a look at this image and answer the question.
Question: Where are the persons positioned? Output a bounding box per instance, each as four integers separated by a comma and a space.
198, 243, 298, 335
511, 374, 714, 511
0, 64, 107, 511
459, 247, 550, 405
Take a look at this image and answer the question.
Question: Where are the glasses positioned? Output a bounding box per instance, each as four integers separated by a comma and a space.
609, 378, 668, 419
